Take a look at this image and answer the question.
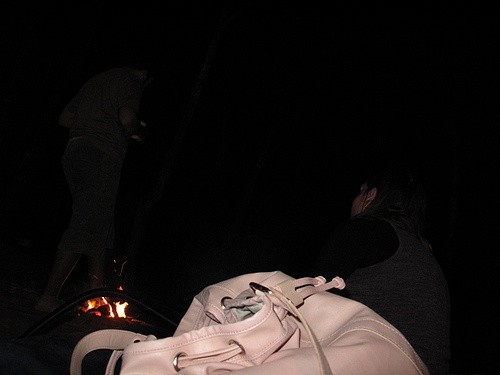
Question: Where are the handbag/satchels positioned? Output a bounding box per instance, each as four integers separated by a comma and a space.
70, 270, 430, 375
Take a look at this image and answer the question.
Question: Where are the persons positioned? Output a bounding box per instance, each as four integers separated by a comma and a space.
34, 55, 164, 314
309, 163, 452, 375
